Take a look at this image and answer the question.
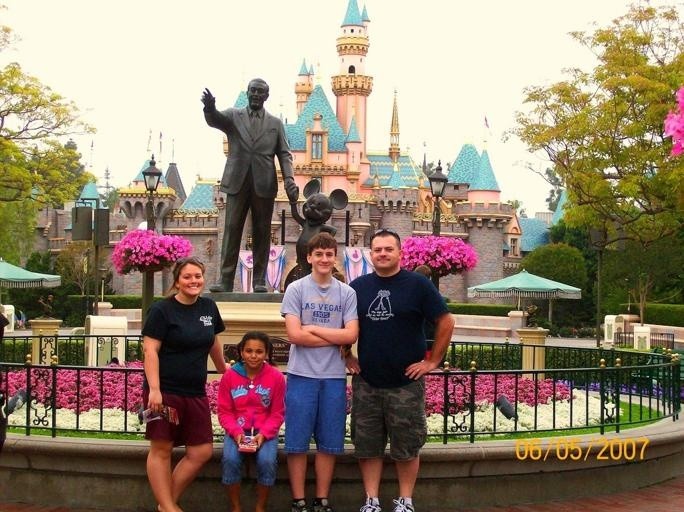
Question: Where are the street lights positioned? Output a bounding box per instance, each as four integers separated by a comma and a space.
427, 159, 448, 292
141, 154, 162, 231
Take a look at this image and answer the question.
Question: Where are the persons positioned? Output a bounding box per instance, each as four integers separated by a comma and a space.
200, 79, 297, 293
218, 332, 286, 512
140, 257, 232, 511
342, 230, 456, 512
280, 232, 359, 512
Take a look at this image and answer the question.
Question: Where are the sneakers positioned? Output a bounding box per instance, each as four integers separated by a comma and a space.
293, 499, 311, 511
358, 494, 382, 512
314, 499, 335, 512
389, 498, 414, 512
